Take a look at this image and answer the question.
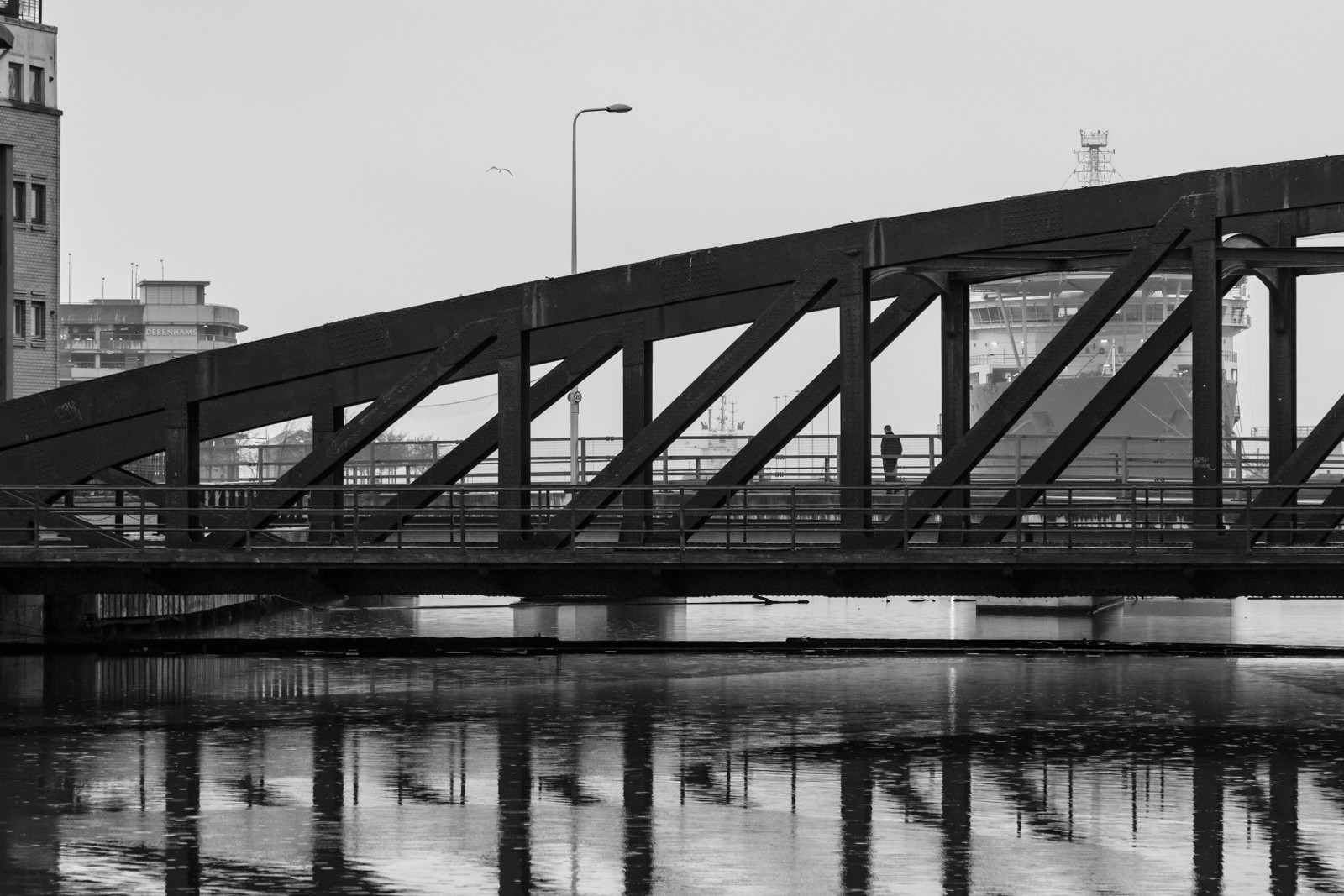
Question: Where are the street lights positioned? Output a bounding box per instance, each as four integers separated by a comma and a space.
772, 390, 801, 476
569, 103, 633, 483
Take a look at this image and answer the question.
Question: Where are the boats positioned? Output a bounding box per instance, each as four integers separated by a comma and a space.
688, 398, 750, 479
963, 126, 1255, 497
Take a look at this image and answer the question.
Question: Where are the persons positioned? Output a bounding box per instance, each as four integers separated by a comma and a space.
881, 425, 902, 494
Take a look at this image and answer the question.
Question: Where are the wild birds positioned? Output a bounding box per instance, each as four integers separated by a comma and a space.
486, 166, 513, 176
